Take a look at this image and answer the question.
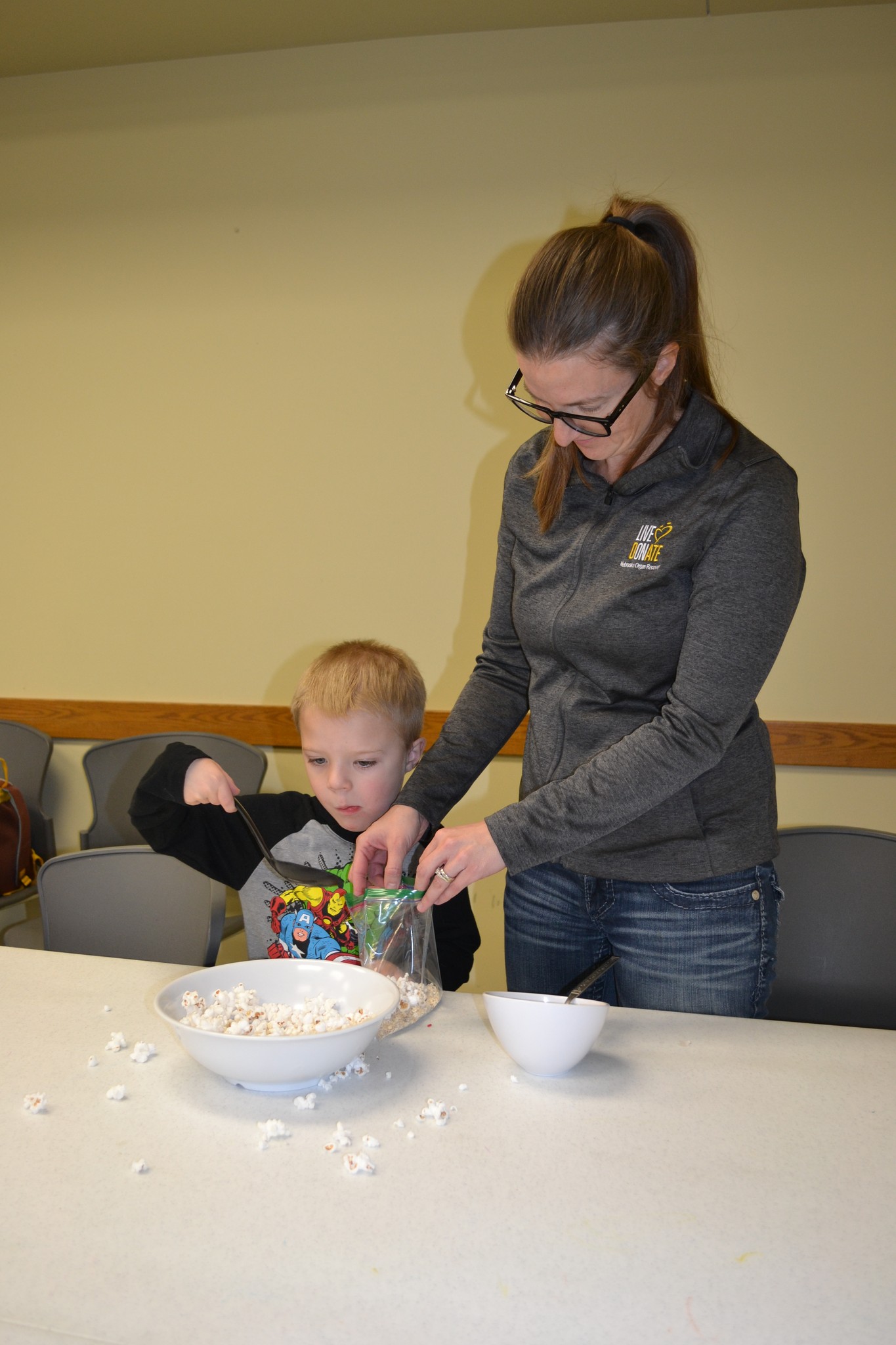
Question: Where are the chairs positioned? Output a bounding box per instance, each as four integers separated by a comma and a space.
762, 826, 895, 1032
35, 843, 228, 969
0, 720, 58, 864
78, 732, 271, 939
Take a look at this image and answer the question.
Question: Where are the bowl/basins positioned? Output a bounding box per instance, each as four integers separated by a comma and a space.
484, 987, 610, 1077
154, 956, 403, 1091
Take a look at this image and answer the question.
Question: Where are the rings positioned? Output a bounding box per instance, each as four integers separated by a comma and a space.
435, 867, 452, 883
440, 866, 454, 880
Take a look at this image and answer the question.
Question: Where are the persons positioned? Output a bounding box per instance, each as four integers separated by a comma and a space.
128, 639, 480, 992
349, 192, 807, 1015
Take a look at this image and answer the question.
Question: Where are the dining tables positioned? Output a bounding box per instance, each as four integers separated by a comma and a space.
0, 947, 895, 1345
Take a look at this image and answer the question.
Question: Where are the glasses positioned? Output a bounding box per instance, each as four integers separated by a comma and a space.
505, 343, 664, 437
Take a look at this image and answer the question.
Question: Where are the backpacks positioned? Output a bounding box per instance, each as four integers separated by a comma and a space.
0, 757, 32, 895
